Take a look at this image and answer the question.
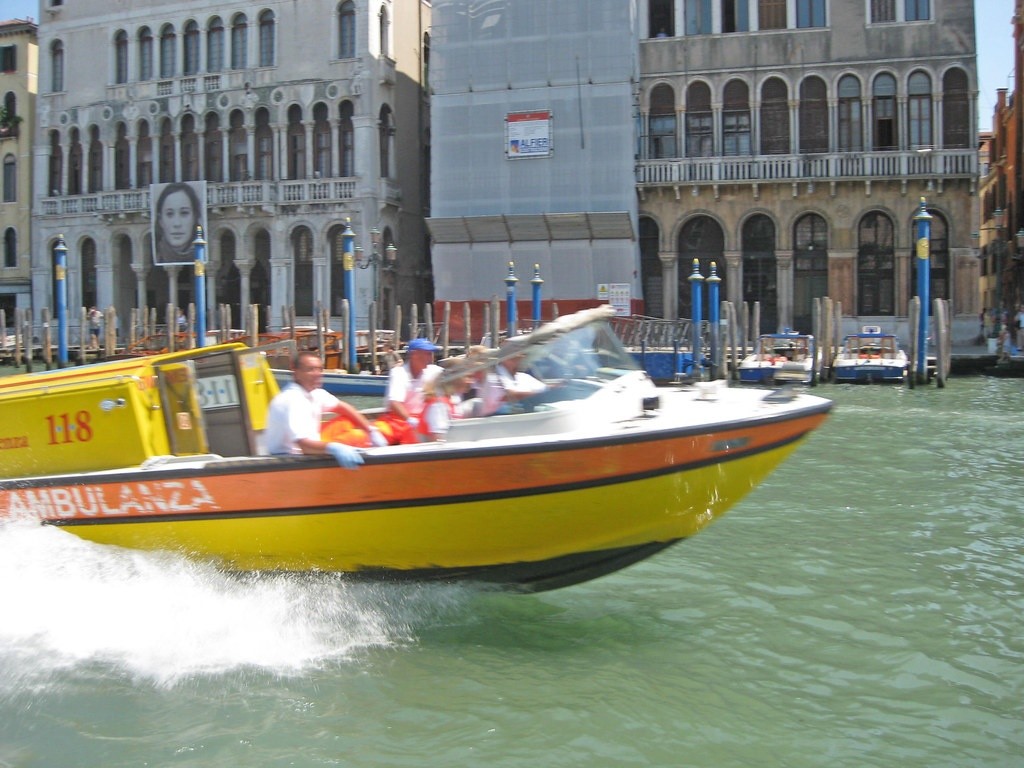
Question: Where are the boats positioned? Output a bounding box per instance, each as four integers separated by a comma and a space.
1, 303, 836, 597
736, 326, 822, 382
831, 324, 911, 383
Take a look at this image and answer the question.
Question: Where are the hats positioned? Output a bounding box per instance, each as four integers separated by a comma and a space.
407, 337, 438, 350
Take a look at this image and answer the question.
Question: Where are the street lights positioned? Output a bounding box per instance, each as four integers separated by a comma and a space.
354, 225, 397, 374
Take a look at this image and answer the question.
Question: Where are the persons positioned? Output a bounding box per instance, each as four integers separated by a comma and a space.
178, 309, 185, 332
155, 183, 205, 263
784, 343, 795, 361
265, 351, 389, 470
87, 305, 104, 350
383, 339, 565, 443
980, 301, 1024, 351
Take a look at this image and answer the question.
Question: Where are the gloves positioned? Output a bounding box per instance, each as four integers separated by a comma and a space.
325, 441, 365, 470
368, 426, 388, 448
407, 417, 419, 426
